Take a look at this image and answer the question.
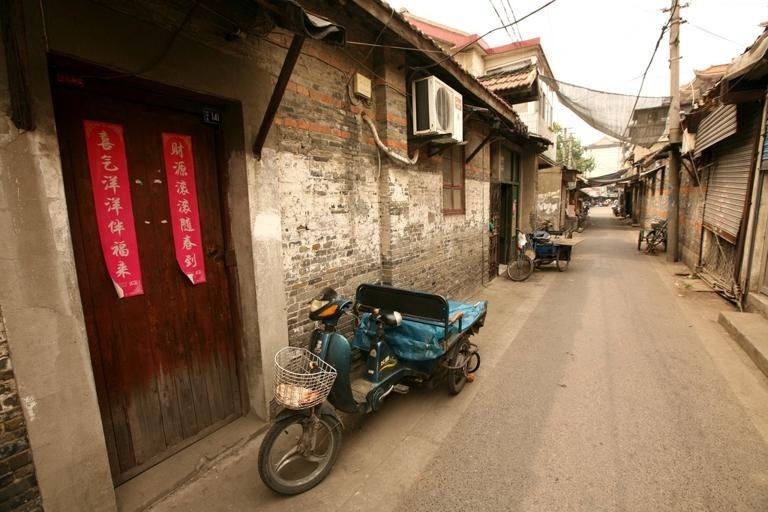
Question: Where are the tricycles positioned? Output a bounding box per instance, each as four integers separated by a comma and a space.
507, 228, 571, 281
259, 283, 488, 495
637, 219, 668, 253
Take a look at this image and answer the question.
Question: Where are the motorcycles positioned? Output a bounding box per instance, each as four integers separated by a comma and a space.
577, 208, 589, 233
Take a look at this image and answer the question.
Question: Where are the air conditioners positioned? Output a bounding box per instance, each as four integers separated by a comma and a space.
412, 75, 465, 147
